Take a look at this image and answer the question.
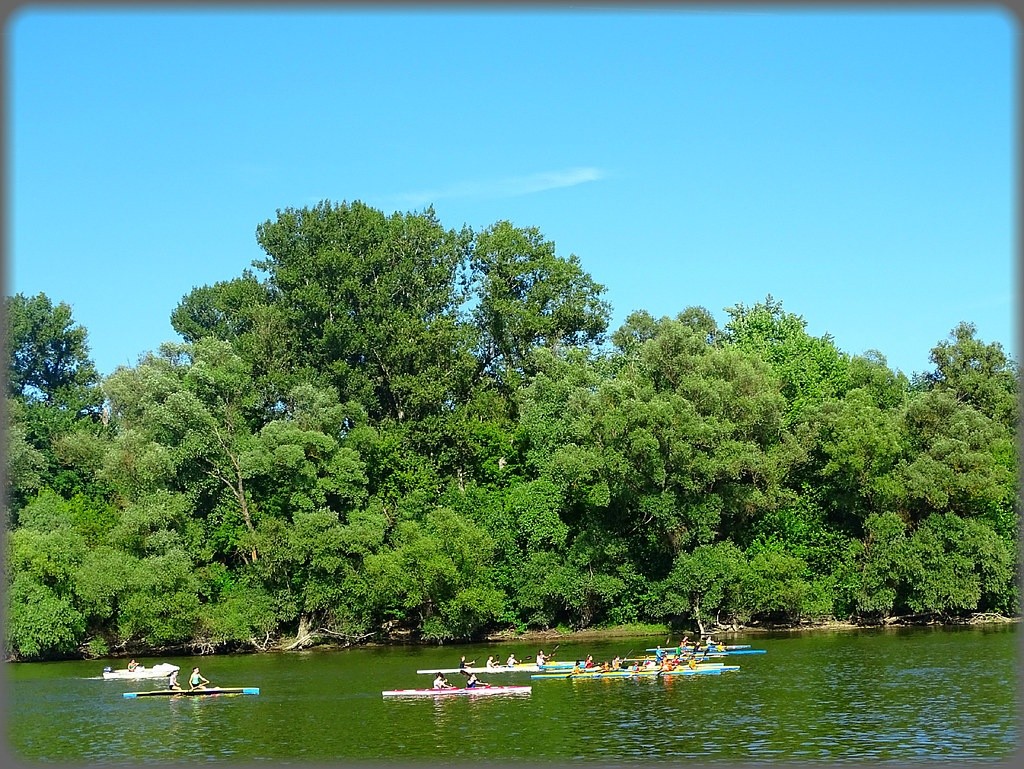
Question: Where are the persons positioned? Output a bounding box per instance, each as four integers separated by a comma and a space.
655, 637, 704, 672
466, 673, 488, 688
434, 672, 453, 688
599, 654, 651, 673
487, 655, 499, 668
507, 653, 522, 665
189, 667, 209, 690
572, 661, 583, 674
718, 640, 726, 652
460, 656, 475, 668
706, 636, 720, 645
585, 655, 594, 668
128, 660, 139, 672
168, 670, 182, 690
537, 649, 551, 665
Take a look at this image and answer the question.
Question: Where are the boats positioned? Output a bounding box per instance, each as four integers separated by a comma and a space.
416, 661, 588, 674
102, 663, 181, 680
122, 686, 260, 699
530, 644, 768, 680
381, 685, 532, 696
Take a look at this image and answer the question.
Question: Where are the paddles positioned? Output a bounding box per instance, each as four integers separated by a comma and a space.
176, 681, 209, 699
130, 662, 139, 672
549, 644, 560, 658
443, 677, 455, 687
496, 654, 500, 661
566, 671, 577, 679
471, 655, 481, 666
594, 636, 720, 670
518, 656, 532, 663
460, 670, 486, 683
